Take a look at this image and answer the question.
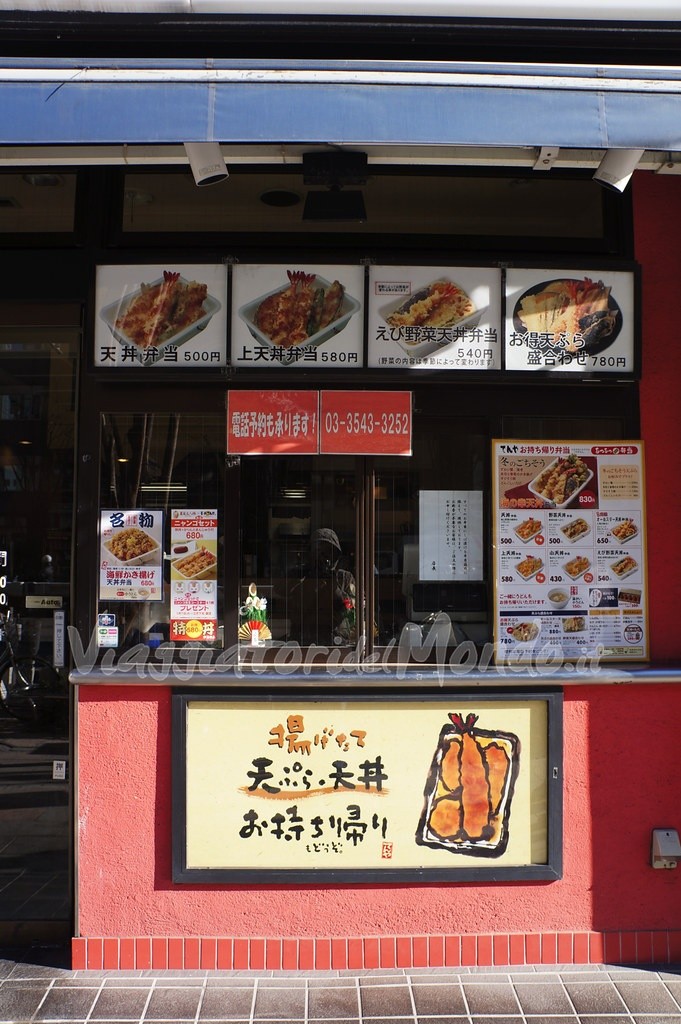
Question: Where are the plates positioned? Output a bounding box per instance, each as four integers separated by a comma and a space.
512, 278, 623, 358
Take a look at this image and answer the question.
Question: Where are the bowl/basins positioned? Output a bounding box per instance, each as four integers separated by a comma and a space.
513, 518, 640, 581
102, 530, 161, 566
171, 549, 217, 581
547, 587, 571, 608
529, 458, 593, 509
98, 276, 489, 368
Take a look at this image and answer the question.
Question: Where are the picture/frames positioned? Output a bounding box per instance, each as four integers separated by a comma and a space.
169, 688, 565, 885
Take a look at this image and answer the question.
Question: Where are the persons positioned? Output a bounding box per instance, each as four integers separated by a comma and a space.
268, 529, 356, 644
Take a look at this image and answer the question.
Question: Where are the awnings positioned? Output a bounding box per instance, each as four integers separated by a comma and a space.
0, 56, 680, 151
